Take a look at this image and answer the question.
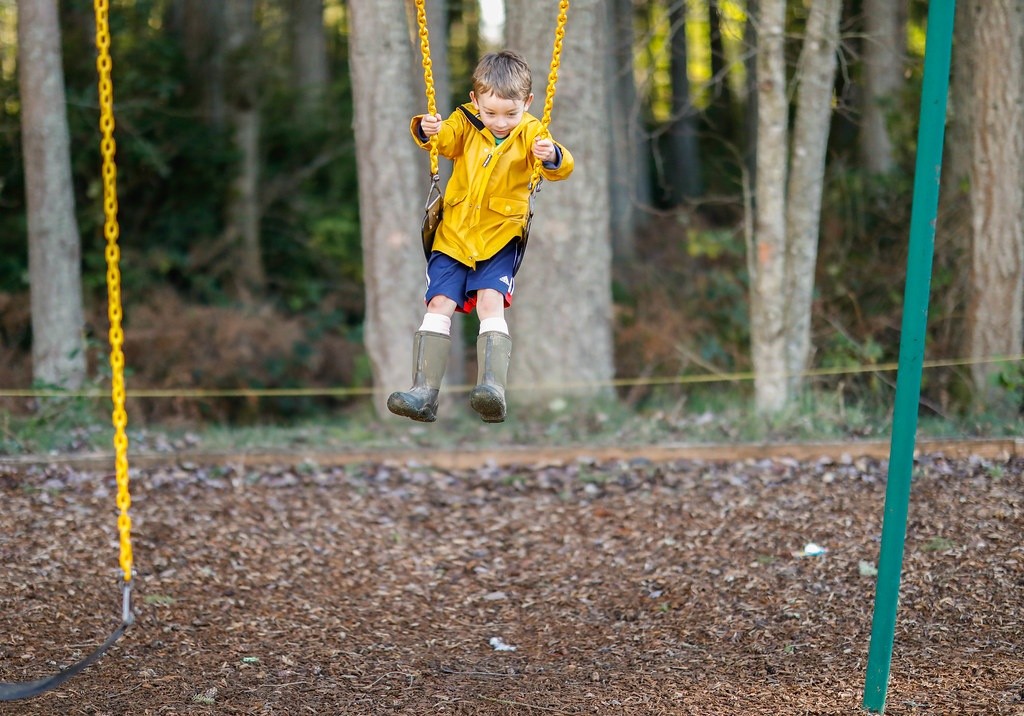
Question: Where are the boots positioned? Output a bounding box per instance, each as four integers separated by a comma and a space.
387, 331, 451, 422
469, 332, 513, 423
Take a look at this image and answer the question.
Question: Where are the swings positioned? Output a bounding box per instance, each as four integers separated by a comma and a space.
414, 0, 570, 278
0, 0, 140, 703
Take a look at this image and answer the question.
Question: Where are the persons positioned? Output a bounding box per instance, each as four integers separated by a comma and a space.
387, 51, 576, 423
